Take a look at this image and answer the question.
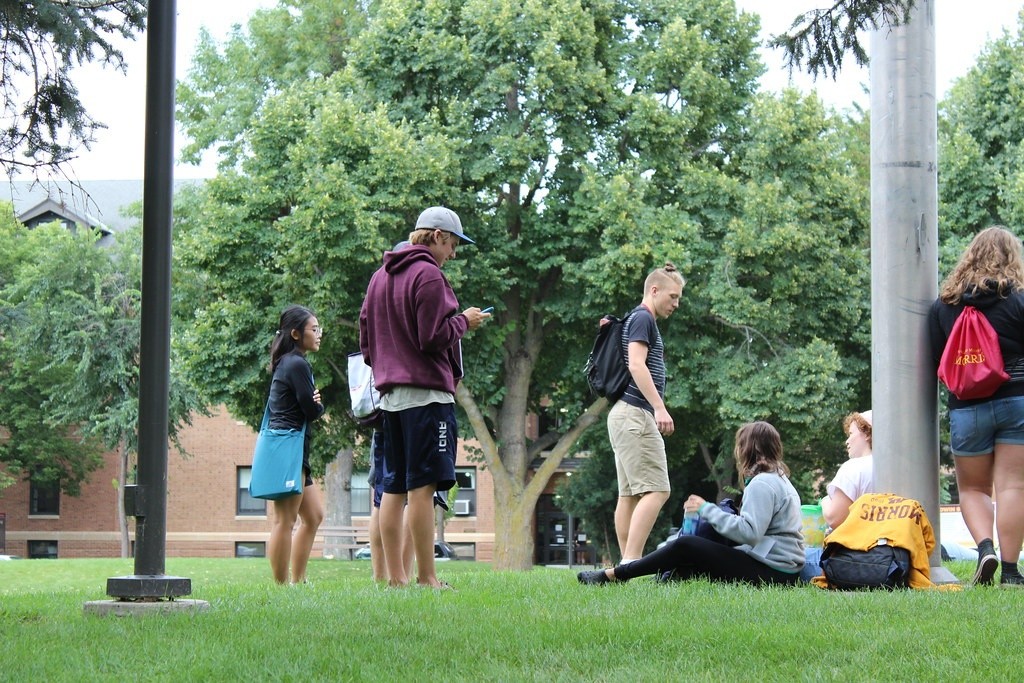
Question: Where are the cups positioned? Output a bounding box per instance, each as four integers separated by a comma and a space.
801, 504, 825, 545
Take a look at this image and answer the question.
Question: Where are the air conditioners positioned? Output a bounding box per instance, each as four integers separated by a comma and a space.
446, 500, 471, 516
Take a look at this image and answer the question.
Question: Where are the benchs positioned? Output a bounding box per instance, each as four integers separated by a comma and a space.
292, 525, 370, 560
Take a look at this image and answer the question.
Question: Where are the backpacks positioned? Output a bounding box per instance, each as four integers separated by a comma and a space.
346, 351, 382, 418
582, 307, 656, 405
819, 541, 911, 592
938, 305, 1011, 400
655, 498, 739, 585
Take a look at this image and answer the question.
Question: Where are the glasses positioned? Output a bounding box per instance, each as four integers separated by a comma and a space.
303, 325, 323, 335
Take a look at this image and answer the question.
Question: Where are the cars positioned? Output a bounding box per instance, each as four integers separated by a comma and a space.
434, 539, 456, 561
354, 543, 372, 561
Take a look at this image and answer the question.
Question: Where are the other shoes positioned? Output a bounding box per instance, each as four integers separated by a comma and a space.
1000, 573, 1024, 587
578, 567, 615, 585
415, 577, 457, 593
973, 547, 998, 586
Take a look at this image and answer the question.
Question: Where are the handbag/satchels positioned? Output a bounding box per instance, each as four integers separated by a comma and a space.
248, 428, 304, 500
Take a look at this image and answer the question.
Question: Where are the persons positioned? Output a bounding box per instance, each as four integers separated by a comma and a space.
250, 307, 324, 584
367, 422, 416, 585
606, 261, 688, 568
929, 227, 1024, 589
360, 207, 491, 591
578, 421, 806, 587
799, 410, 873, 584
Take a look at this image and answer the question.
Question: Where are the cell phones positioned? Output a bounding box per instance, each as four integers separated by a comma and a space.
481, 307, 494, 314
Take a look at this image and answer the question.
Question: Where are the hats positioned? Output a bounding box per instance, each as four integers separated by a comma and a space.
415, 206, 476, 246
860, 409, 872, 427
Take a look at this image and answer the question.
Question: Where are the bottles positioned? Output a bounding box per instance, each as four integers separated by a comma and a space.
682, 510, 700, 535
